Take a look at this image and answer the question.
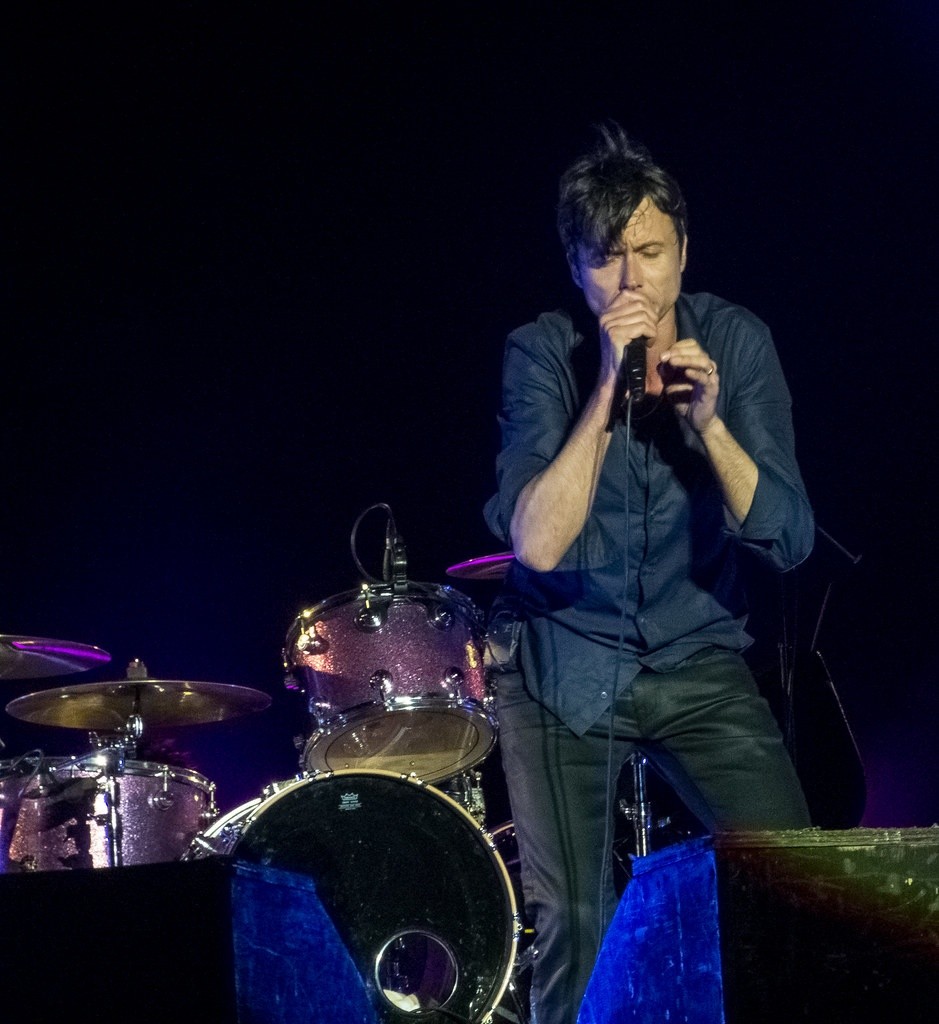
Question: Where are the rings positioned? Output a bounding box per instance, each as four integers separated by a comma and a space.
707, 362, 715, 376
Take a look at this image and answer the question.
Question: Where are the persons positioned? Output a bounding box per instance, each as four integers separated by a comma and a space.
481, 120, 812, 1024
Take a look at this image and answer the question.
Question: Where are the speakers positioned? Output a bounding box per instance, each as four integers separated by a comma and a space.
570, 828, 939, 1023
0, 854, 379, 1024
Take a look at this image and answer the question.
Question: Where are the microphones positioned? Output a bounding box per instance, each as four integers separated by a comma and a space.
381, 519, 395, 581
622, 336, 648, 405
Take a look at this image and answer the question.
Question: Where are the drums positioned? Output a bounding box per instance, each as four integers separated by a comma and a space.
281, 578, 499, 784
0, 756, 218, 874
182, 769, 518, 1024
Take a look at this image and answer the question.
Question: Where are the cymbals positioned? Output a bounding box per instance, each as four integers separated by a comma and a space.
0, 632, 111, 680
445, 549, 515, 578
6, 680, 273, 731
